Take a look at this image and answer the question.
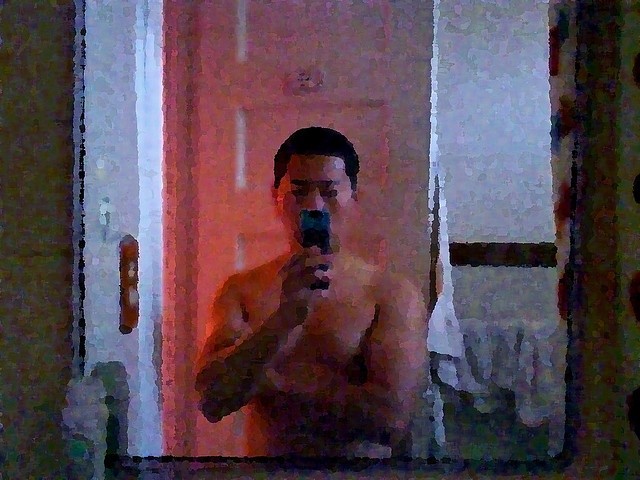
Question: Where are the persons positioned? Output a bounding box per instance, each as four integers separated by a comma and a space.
193, 127, 428, 457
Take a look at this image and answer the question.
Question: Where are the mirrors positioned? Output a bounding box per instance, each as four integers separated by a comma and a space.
72, 0, 584, 472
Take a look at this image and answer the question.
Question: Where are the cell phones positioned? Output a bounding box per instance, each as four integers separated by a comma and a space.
299, 208, 331, 290
